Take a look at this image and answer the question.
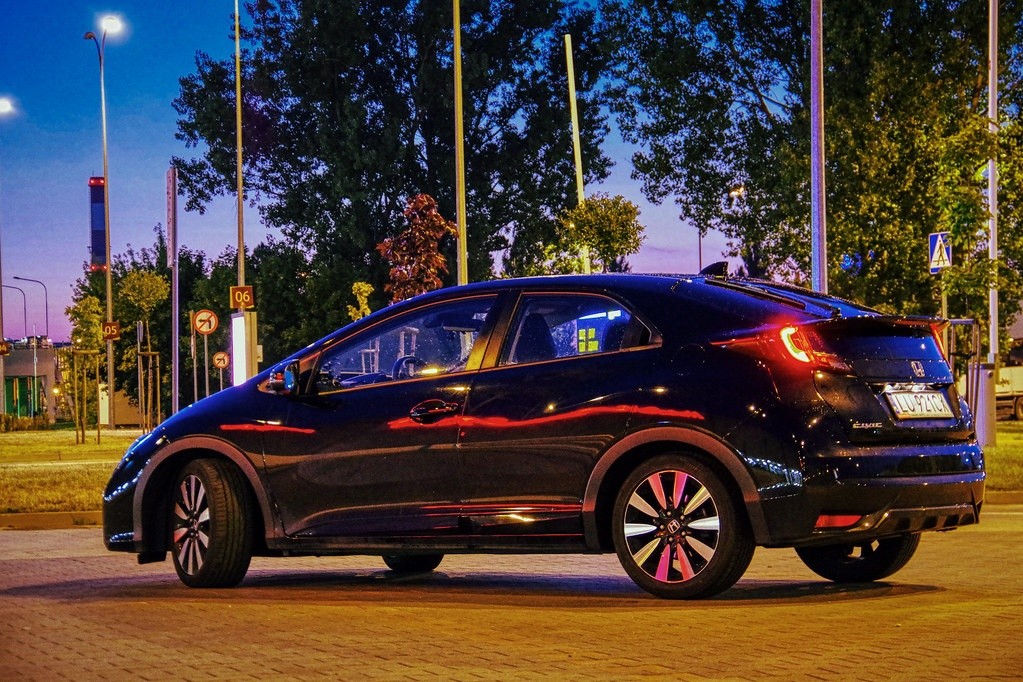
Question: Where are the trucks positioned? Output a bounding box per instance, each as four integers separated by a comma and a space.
954, 364, 1023, 422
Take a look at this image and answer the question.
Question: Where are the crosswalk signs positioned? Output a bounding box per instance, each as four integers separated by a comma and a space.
929, 231, 953, 275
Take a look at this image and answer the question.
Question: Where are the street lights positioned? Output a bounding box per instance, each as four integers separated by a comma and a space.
13, 276, 50, 350
83, 15, 123, 431
698, 184, 745, 272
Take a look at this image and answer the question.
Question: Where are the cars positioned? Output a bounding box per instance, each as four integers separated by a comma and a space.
99, 268, 986, 601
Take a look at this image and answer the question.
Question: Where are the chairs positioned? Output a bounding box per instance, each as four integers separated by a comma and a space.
601, 318, 648, 353
514, 312, 559, 364
